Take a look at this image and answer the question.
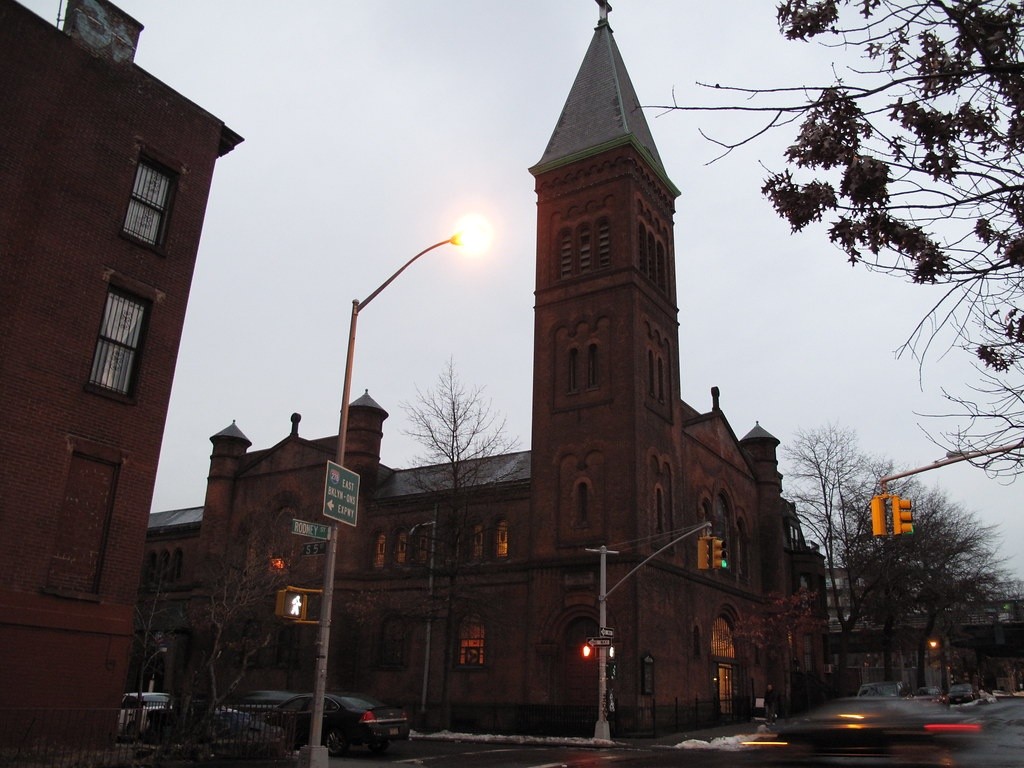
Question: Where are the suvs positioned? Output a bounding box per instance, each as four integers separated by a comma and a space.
857, 680, 911, 700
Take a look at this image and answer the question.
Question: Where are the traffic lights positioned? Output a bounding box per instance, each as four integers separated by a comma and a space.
891, 494, 914, 536
712, 538, 728, 569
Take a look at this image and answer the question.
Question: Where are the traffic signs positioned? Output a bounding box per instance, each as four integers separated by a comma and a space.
292, 518, 332, 542
322, 458, 361, 527
301, 542, 327, 556
585, 637, 612, 648
599, 626, 614, 637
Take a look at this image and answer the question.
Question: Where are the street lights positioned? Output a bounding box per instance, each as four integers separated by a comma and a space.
305, 233, 465, 768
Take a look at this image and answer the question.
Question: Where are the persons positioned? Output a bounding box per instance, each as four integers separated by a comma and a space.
763, 684, 779, 726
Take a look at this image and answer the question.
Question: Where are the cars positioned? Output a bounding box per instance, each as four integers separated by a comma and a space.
946, 683, 981, 704
912, 685, 949, 706
255, 692, 414, 758
187, 700, 252, 742
117, 692, 178, 740
